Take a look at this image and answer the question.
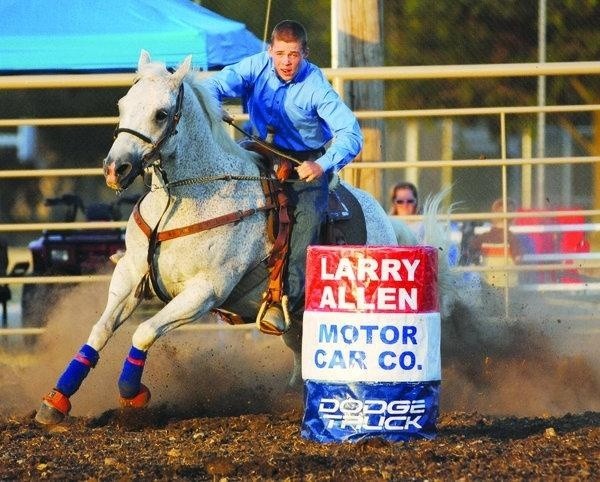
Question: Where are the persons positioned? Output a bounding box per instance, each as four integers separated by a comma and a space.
389, 182, 418, 216
469, 198, 523, 316
199, 20, 364, 334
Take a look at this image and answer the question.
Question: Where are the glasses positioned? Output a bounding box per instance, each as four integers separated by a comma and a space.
393, 197, 417, 205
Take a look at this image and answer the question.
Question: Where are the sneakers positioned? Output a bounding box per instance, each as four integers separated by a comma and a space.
259, 305, 286, 332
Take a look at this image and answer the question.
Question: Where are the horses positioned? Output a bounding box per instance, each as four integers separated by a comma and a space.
32, 46, 467, 428
